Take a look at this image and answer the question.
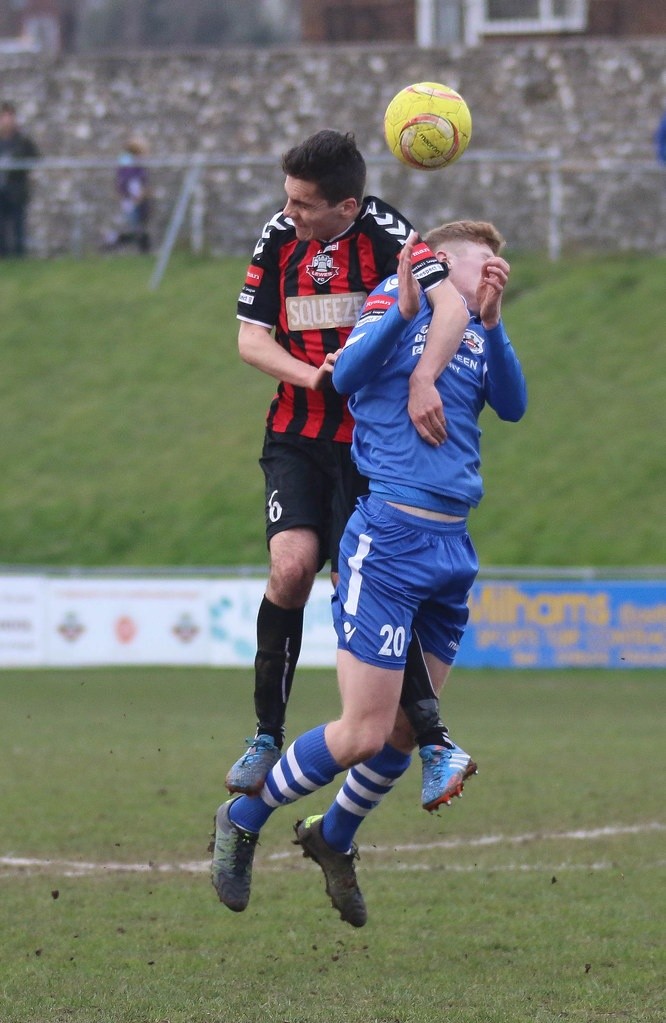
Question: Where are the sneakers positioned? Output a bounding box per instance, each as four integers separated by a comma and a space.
291, 814, 368, 928
224, 734, 282, 796
209, 794, 261, 912
418, 742, 479, 814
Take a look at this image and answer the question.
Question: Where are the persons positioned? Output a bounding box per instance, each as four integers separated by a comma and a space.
0, 101, 39, 258
115, 141, 160, 255
224, 130, 479, 817
209, 218, 527, 929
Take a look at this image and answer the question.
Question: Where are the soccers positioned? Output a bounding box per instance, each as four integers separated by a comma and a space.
382, 79, 474, 169
